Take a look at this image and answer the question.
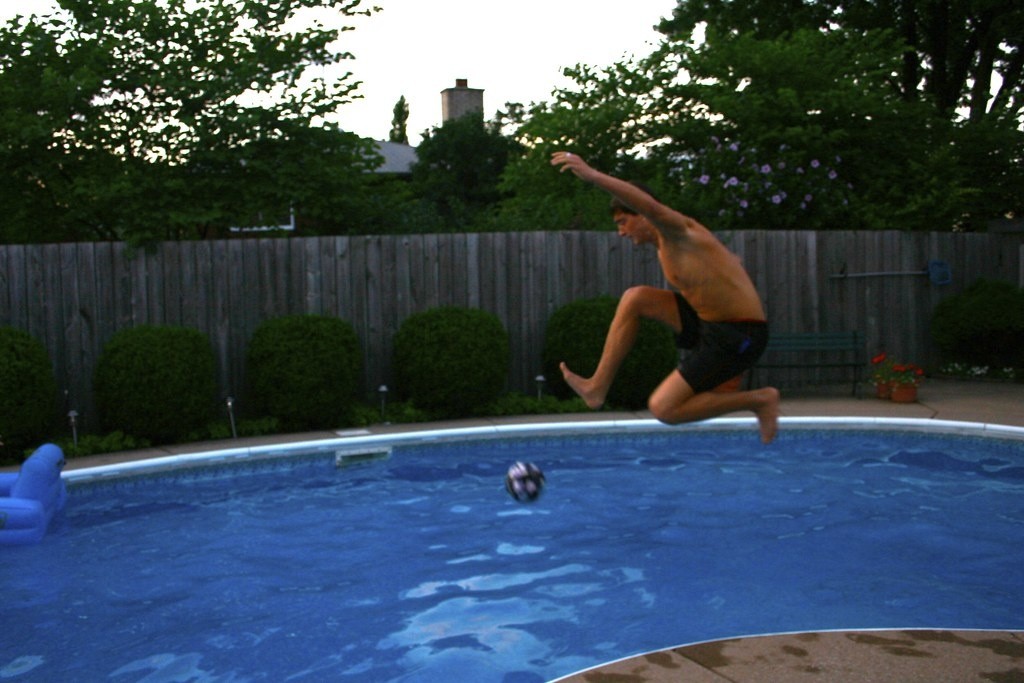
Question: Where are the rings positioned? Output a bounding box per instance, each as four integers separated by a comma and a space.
566, 153, 570, 157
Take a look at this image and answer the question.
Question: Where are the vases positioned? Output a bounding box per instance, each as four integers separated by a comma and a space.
875, 378, 917, 402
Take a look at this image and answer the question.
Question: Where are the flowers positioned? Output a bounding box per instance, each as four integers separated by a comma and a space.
869, 352, 925, 385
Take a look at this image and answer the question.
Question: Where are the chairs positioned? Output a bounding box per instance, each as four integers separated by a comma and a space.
0, 442, 70, 546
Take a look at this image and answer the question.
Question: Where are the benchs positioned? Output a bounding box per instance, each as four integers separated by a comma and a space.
746, 330, 867, 397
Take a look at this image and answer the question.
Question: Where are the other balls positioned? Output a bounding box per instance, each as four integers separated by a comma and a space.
506, 459, 543, 503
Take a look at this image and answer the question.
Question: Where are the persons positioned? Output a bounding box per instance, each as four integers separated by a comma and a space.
551, 152, 779, 444
